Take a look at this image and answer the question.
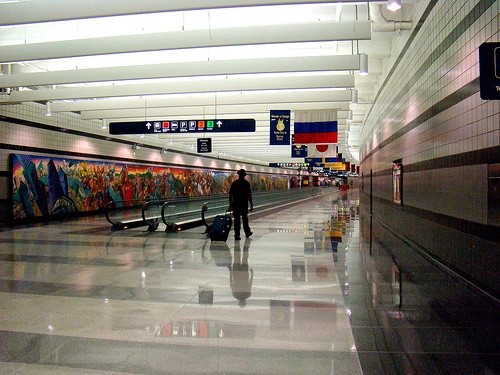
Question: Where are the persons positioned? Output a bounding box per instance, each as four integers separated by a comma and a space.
228, 168, 253, 240
228, 241, 255, 309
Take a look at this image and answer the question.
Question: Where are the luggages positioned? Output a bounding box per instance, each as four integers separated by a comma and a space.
206, 210, 233, 242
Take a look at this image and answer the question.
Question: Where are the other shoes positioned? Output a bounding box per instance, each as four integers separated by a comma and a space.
235, 237, 241, 240
246, 232, 253, 238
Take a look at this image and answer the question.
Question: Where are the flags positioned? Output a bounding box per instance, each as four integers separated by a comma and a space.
294, 110, 359, 173
303, 205, 359, 263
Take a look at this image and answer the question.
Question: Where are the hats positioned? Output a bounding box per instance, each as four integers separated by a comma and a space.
237, 169, 248, 176
